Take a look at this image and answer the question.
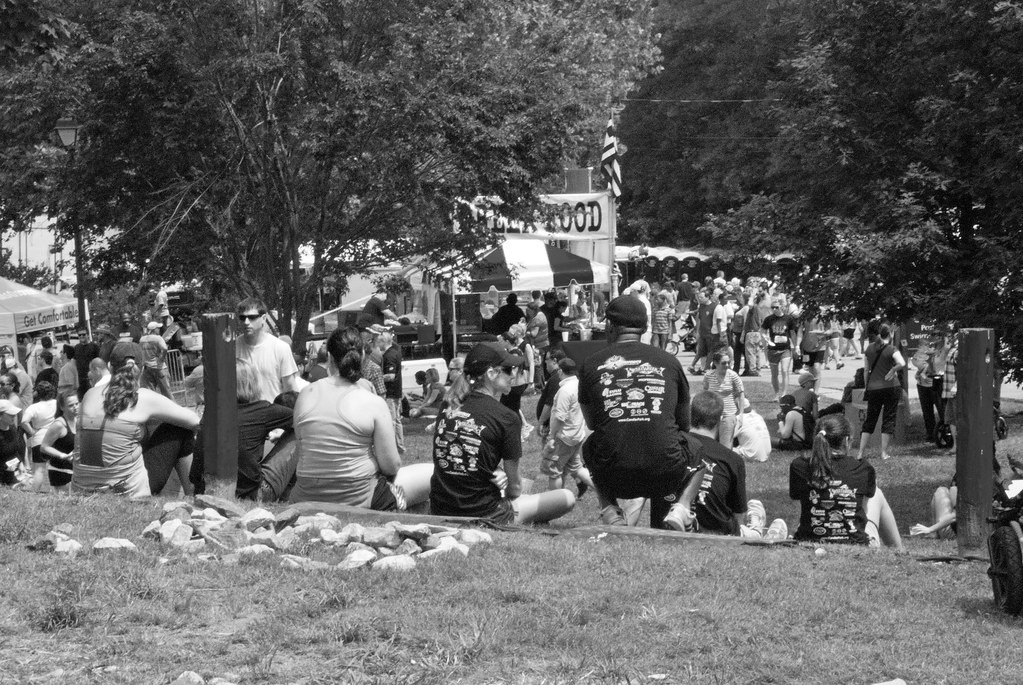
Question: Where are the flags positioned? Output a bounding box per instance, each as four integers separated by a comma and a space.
600, 113, 621, 199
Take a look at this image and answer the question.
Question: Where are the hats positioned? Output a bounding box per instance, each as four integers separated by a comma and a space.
462, 341, 529, 374
109, 341, 144, 366
605, 294, 647, 327
798, 373, 818, 386
147, 321, 163, 334
2, 358, 16, 370
92, 324, 111, 336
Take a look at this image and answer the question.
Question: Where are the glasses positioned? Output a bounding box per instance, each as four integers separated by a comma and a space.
0, 354, 5, 359
239, 313, 262, 321
77, 333, 87, 337
0, 381, 11, 387
720, 360, 731, 365
770, 306, 779, 309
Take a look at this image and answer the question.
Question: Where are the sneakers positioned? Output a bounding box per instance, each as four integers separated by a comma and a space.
743, 501, 767, 539
666, 503, 701, 532
765, 518, 790, 542
599, 506, 629, 526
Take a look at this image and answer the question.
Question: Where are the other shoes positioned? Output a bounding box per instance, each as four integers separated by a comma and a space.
739, 369, 759, 377
576, 480, 588, 498
856, 452, 865, 460
520, 423, 536, 443
687, 366, 706, 375
836, 362, 845, 369
881, 452, 890, 460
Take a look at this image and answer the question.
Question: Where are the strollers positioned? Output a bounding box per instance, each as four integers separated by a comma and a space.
665, 312, 698, 355
934, 398, 1009, 449
987, 472, 1023, 615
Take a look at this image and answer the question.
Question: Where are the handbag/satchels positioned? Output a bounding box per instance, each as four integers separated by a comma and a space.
862, 390, 869, 402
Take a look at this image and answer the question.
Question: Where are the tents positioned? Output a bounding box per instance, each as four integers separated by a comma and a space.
0, 276, 91, 357
421, 238, 609, 357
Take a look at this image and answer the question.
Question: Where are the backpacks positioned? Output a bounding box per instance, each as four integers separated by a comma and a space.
783, 407, 815, 448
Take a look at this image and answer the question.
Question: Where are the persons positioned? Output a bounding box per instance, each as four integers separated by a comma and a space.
910, 485, 957, 540
0, 400, 25, 490
577, 294, 706, 534
650, 391, 788, 541
0, 265, 1023, 502
430, 341, 576, 525
70, 341, 202, 499
789, 413, 903, 548
189, 358, 299, 501
290, 326, 433, 512
20, 380, 57, 490
40, 389, 79, 490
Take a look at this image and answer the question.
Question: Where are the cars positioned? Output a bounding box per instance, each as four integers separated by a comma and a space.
166, 278, 205, 317
